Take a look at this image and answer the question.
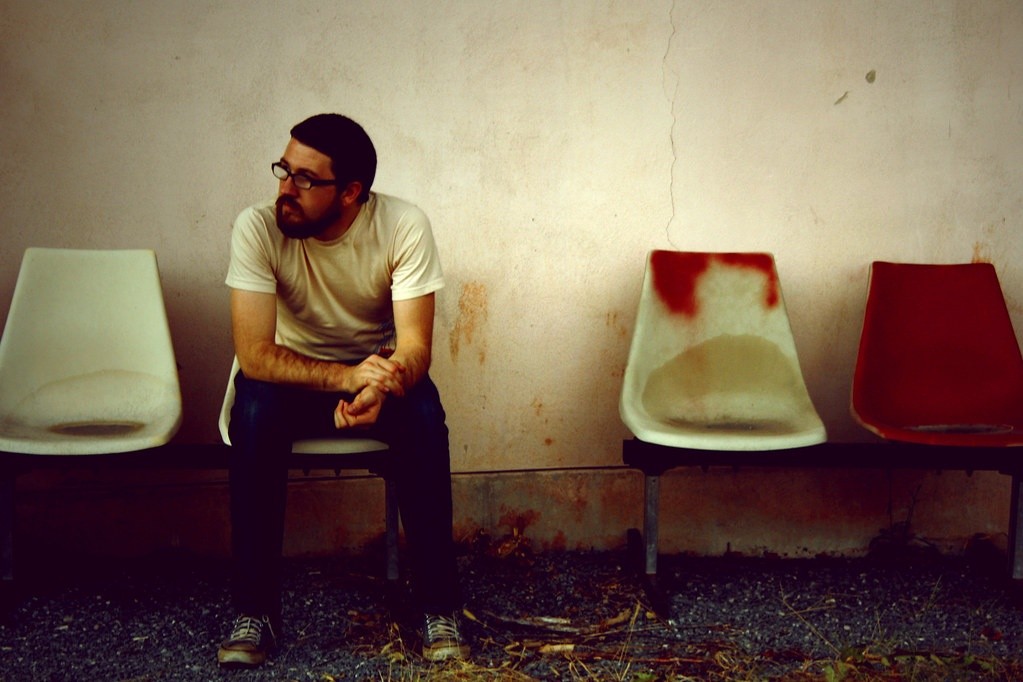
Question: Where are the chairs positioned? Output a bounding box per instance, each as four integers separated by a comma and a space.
849, 261, 1023, 582
619, 248, 826, 591
218, 352, 397, 583
0, 249, 184, 452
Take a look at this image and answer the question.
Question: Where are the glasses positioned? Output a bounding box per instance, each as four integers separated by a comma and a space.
272, 162, 353, 190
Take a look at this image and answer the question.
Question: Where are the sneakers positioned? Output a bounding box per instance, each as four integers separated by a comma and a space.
422, 612, 470, 662
218, 611, 281, 664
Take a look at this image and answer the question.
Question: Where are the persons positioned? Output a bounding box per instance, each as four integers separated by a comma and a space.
216, 112, 472, 667
